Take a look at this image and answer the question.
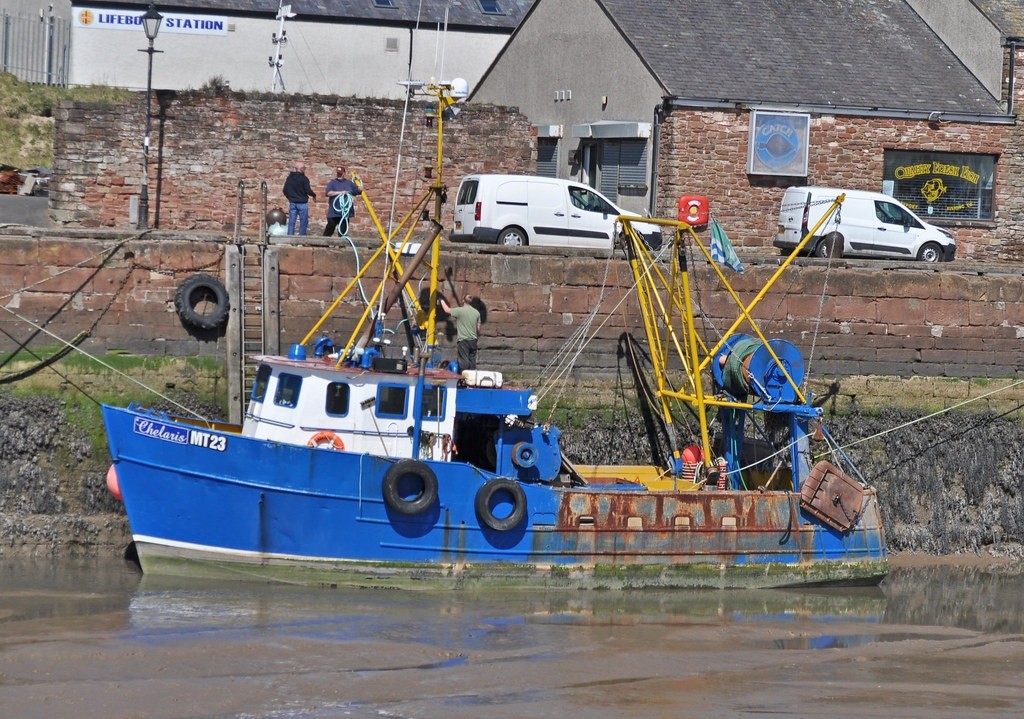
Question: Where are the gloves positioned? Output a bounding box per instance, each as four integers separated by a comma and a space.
438, 296, 443, 304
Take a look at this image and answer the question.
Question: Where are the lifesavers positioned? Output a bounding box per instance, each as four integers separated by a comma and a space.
474, 477, 526, 531
175, 273, 230, 331
308, 432, 345, 451
382, 458, 438, 515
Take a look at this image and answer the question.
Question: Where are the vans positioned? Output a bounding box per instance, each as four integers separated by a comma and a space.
448, 174, 664, 260
773, 185, 957, 264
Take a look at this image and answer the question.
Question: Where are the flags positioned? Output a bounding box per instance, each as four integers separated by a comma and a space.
711, 219, 746, 274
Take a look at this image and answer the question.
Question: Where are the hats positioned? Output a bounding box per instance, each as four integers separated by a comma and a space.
337, 166, 346, 174
464, 294, 472, 302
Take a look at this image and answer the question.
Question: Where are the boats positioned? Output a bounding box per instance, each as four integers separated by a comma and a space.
91, 78, 906, 588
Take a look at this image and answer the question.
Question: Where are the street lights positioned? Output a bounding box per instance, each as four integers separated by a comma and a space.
136, 3, 165, 234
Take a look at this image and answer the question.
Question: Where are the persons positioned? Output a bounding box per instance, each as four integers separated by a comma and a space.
323, 166, 363, 237
438, 293, 481, 374
282, 163, 316, 236
